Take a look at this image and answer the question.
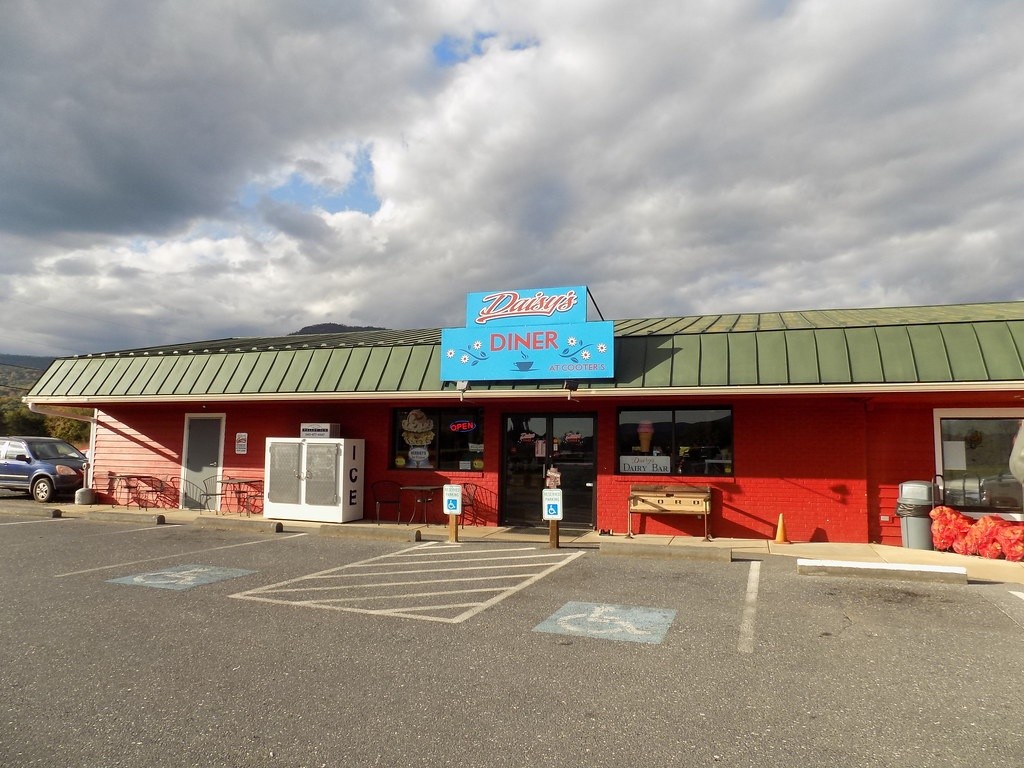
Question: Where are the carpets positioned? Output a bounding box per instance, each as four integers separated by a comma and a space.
502, 526, 590, 537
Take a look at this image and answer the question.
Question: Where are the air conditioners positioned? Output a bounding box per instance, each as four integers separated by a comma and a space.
300, 423, 341, 438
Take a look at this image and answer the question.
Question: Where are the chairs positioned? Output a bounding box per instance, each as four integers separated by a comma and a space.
443, 482, 478, 529
88, 474, 169, 511
200, 474, 264, 518
372, 480, 402, 526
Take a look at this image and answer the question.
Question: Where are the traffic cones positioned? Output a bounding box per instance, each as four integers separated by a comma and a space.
773, 513, 792, 545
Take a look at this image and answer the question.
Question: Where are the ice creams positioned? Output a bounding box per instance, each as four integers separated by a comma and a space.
401, 409, 433, 467
637, 418, 654, 452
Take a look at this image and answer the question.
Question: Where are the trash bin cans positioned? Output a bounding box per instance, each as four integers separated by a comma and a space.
897, 480, 943, 550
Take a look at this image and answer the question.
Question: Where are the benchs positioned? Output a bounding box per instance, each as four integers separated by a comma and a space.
625, 485, 713, 542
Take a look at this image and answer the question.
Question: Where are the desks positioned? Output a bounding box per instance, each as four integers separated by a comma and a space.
221, 479, 262, 516
400, 485, 443, 527
122, 475, 153, 508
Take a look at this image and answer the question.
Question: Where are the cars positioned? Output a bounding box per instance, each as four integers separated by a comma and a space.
688, 446, 724, 475
547, 451, 595, 491
978, 474, 1023, 509
0, 436, 89, 504
